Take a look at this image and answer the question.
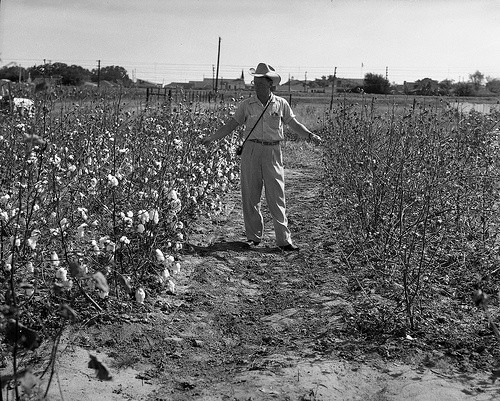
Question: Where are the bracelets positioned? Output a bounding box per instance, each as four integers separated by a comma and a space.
309, 132, 312, 140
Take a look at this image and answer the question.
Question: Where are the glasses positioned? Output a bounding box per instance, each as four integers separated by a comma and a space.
251, 80, 270, 86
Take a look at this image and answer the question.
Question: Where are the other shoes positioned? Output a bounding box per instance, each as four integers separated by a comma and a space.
244, 240, 259, 246
279, 243, 299, 251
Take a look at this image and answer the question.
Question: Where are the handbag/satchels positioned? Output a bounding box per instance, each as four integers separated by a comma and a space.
236, 146, 243, 155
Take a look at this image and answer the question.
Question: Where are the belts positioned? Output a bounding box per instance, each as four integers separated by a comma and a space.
249, 139, 280, 145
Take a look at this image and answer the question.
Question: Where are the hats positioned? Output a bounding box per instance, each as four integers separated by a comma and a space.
248, 63, 281, 86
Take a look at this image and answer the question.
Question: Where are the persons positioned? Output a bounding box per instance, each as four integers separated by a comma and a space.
197, 63, 322, 252
